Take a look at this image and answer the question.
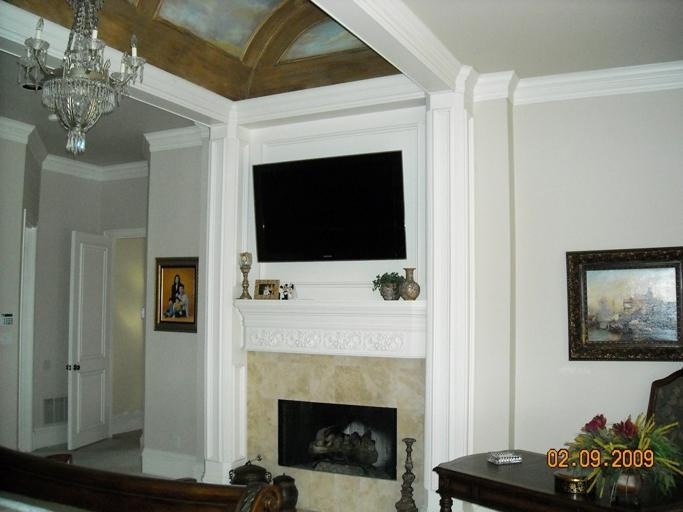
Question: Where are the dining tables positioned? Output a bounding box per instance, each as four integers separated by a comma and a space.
371, 272, 405, 300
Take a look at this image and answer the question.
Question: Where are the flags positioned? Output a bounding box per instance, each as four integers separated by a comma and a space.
399, 267, 420, 301
616, 471, 640, 500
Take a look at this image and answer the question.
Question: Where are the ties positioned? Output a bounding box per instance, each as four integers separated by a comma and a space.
251, 149, 412, 262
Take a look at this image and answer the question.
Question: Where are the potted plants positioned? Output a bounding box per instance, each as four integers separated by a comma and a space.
252, 279, 279, 300
152, 257, 198, 333
565, 247, 682, 362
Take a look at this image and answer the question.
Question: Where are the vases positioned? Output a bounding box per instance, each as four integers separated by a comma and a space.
558, 412, 682, 507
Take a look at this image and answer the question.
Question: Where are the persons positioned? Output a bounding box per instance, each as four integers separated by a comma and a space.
173, 284, 189, 317
170, 274, 183, 302
173, 297, 184, 316
163, 298, 174, 317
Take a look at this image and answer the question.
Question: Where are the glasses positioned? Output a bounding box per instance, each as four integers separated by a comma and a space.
14, 1, 146, 156
